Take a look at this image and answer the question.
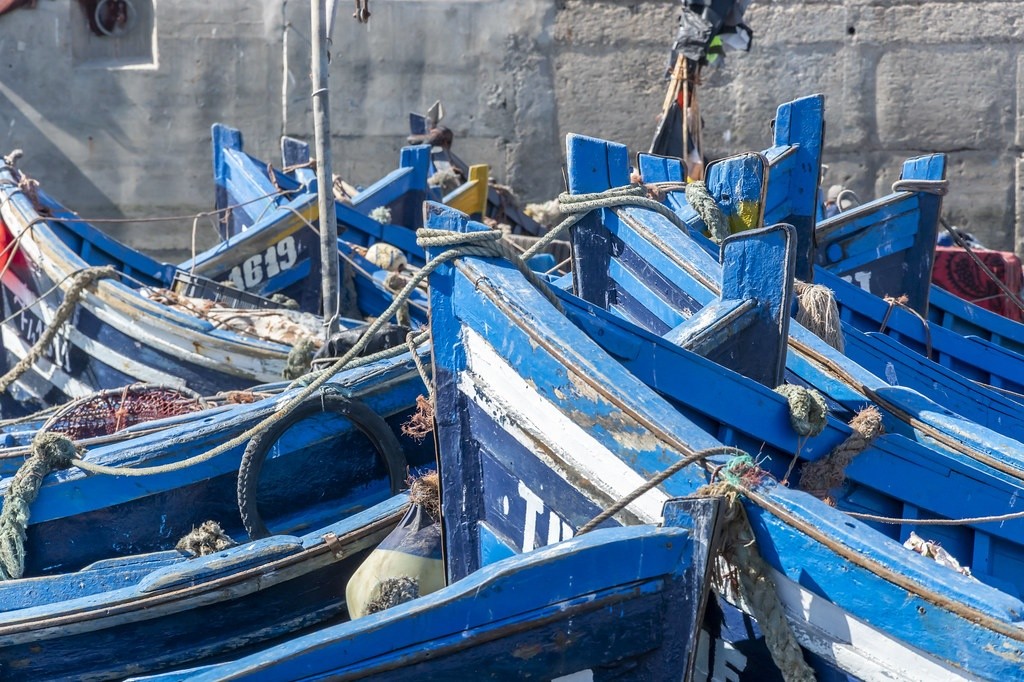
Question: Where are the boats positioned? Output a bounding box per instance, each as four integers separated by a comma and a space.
0, 1, 1023, 682
